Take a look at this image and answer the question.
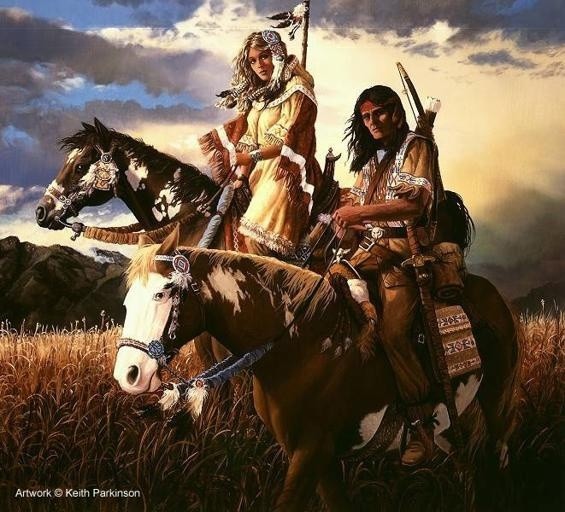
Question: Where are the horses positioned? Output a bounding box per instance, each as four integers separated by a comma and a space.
33, 115, 521, 512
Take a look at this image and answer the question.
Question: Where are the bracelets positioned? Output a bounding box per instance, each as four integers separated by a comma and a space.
249, 150, 264, 163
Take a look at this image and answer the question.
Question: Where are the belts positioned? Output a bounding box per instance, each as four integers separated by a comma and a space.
366, 226, 408, 239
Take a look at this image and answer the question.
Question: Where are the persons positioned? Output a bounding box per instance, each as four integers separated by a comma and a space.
210, 31, 317, 258
326, 84, 443, 472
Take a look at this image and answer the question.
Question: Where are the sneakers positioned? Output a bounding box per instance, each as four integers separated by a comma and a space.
401, 428, 433, 467
